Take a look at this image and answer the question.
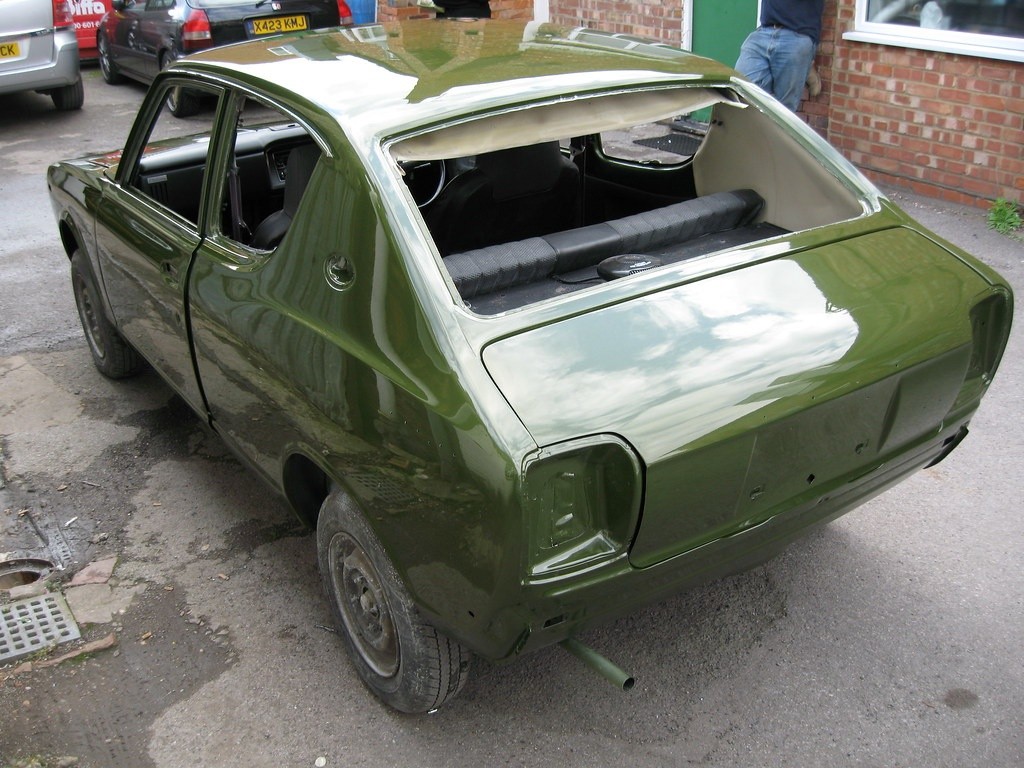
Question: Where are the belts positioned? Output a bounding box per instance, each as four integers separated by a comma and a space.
762, 24, 783, 28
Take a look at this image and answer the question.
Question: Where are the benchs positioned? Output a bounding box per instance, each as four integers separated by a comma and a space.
443, 189, 766, 296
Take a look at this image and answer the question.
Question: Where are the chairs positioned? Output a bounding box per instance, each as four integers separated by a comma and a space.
251, 145, 320, 249
441, 142, 578, 241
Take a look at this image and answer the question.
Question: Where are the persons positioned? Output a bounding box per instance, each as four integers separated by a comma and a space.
734, 0, 826, 112
433, 0, 491, 18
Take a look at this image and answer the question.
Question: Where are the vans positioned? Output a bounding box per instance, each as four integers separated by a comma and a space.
0, 0, 85, 111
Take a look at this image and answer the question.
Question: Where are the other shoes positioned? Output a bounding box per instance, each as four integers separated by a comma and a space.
807, 59, 821, 96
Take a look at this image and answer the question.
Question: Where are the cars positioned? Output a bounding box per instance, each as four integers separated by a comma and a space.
96, 0, 354, 118
46, 18, 1014, 713
